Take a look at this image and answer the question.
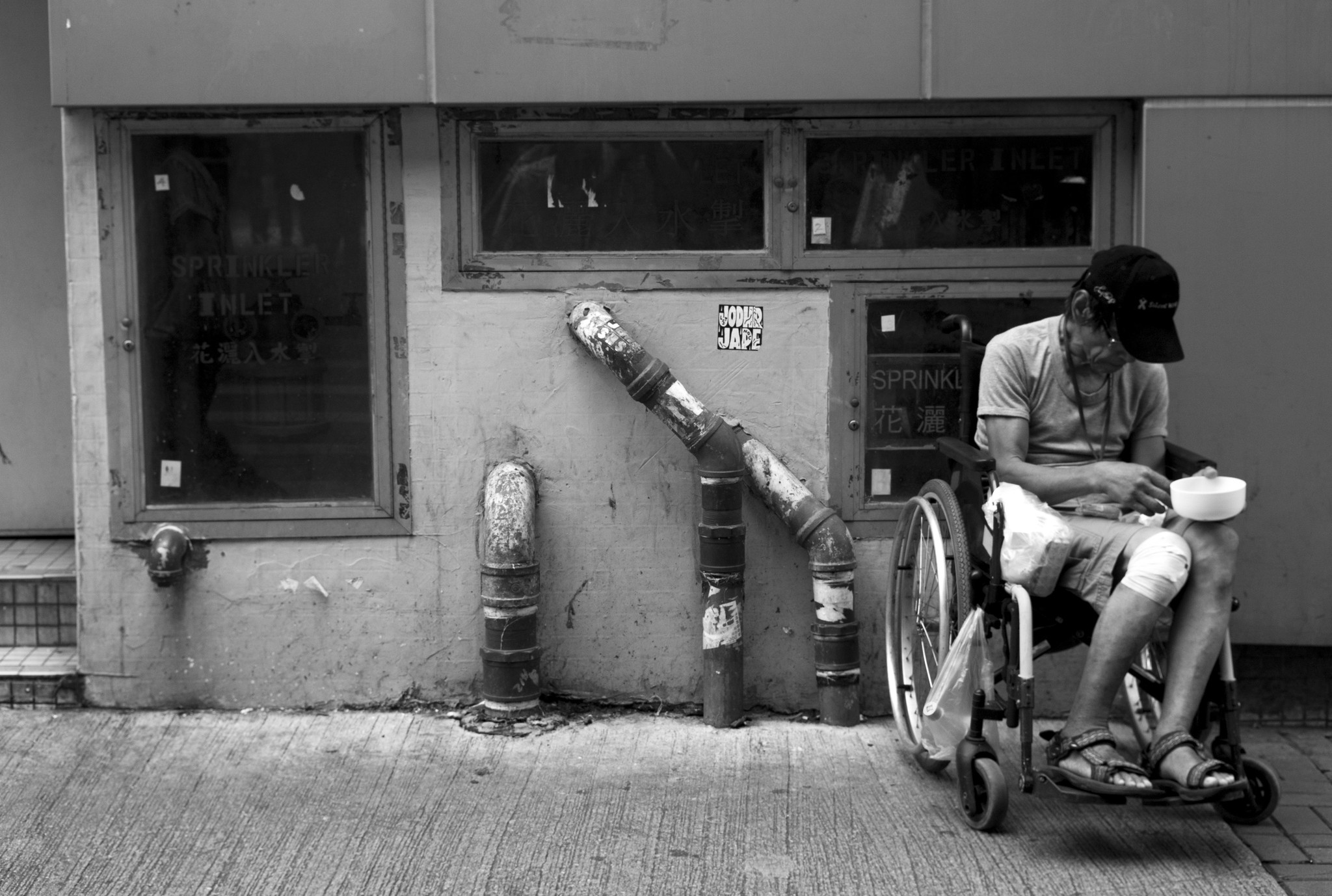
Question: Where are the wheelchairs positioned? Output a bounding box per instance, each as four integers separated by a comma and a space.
884, 437, 1281, 834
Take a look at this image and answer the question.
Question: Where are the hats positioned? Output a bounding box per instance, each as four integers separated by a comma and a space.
1072, 244, 1186, 364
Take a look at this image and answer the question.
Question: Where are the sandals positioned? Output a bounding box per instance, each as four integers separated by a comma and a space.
1045, 728, 1164, 797
1136, 730, 1245, 801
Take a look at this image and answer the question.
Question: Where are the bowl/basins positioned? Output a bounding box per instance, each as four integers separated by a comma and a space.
1169, 475, 1247, 521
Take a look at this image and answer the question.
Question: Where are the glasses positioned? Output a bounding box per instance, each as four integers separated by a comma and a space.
1102, 320, 1122, 349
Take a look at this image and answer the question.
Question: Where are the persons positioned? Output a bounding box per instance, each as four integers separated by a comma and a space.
973, 243, 1248, 802
